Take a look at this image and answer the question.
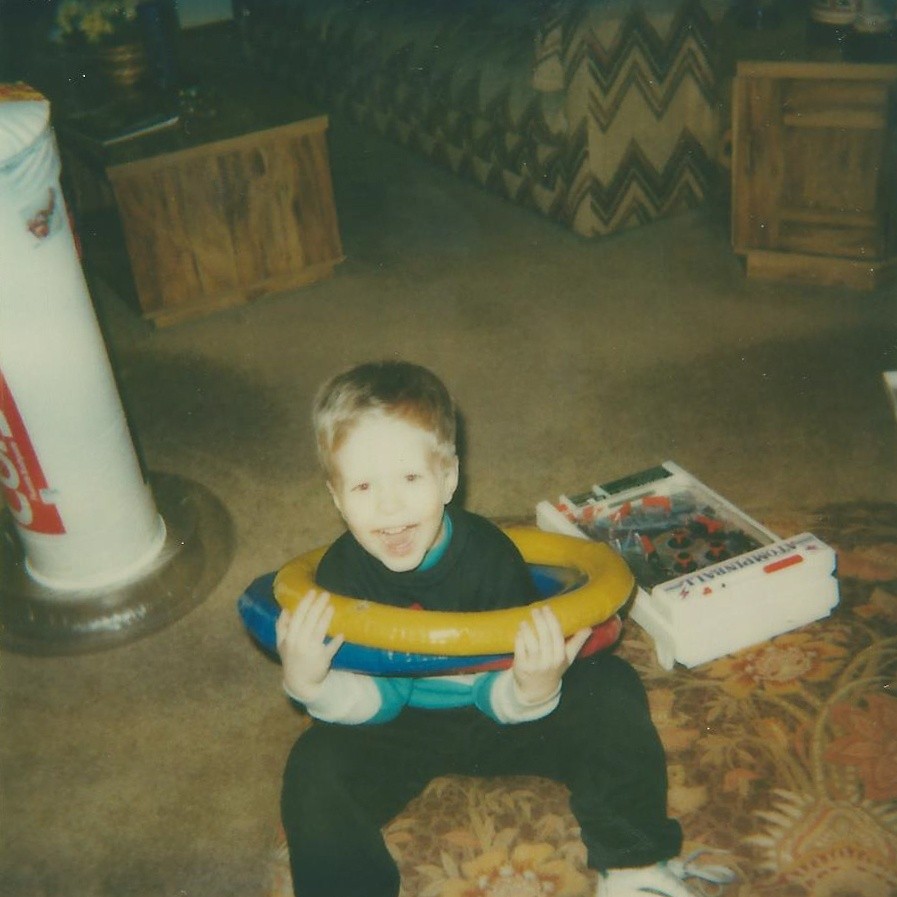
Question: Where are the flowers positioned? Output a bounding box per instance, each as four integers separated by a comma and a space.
55, 2, 139, 51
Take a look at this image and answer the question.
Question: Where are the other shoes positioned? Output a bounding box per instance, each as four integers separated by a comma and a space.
592, 859, 693, 897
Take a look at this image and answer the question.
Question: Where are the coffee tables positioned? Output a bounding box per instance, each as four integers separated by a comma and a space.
59, 54, 348, 328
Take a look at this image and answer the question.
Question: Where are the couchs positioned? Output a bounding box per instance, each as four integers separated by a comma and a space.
263, 0, 769, 245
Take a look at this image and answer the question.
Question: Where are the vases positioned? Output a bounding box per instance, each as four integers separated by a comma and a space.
100, 35, 151, 88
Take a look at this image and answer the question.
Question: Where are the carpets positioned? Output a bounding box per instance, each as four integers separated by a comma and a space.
265, 499, 897, 897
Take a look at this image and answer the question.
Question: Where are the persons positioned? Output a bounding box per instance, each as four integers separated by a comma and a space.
276, 358, 708, 897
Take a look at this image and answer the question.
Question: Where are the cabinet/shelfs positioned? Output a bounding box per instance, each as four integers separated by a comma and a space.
726, 32, 897, 292
